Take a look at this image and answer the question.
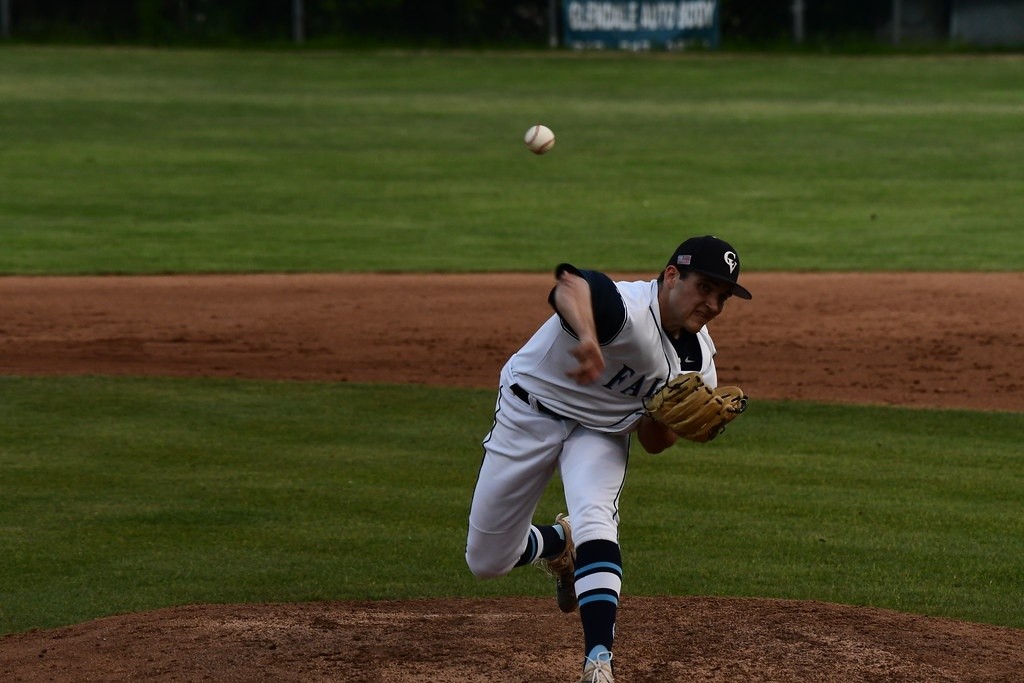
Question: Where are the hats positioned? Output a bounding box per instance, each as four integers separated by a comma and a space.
667, 236, 752, 299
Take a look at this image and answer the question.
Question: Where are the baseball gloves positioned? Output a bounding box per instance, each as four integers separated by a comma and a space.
634, 369, 751, 447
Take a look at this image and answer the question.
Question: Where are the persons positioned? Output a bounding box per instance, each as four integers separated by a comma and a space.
465, 234, 753, 683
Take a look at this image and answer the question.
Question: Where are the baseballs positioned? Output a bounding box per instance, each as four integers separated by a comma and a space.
524, 123, 557, 156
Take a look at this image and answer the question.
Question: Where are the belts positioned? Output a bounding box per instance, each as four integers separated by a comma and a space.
510, 383, 575, 420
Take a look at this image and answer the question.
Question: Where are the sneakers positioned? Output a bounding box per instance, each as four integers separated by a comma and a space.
579, 651, 614, 683
534, 513, 578, 613
